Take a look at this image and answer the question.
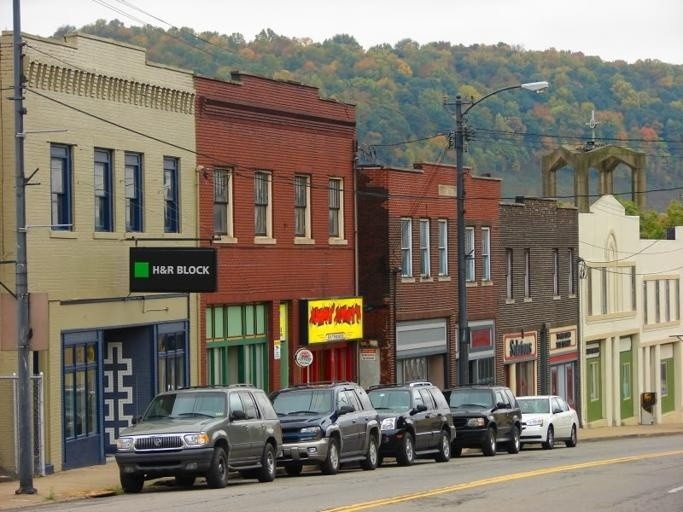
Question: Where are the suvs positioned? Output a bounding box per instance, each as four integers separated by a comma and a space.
266, 378, 383, 474
111, 379, 285, 496
440, 383, 525, 457
364, 379, 460, 467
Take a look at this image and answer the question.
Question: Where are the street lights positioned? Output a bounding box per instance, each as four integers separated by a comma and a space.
452, 75, 552, 384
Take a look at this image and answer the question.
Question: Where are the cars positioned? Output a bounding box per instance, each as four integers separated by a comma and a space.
511, 393, 582, 452
